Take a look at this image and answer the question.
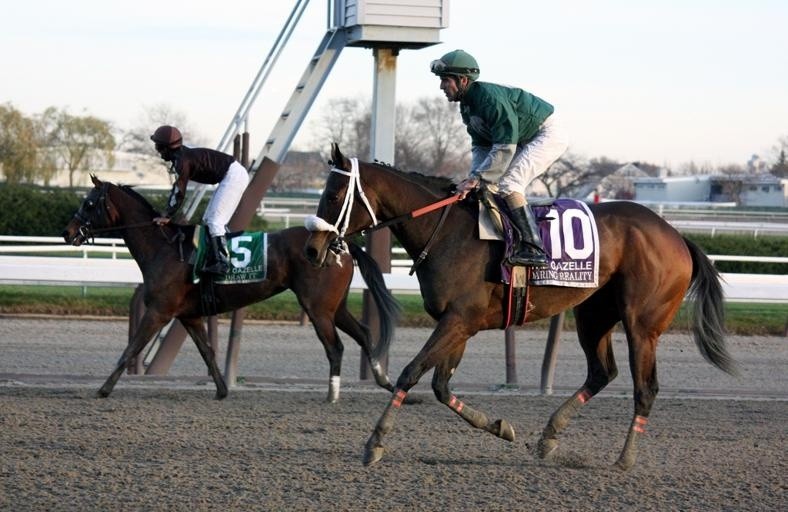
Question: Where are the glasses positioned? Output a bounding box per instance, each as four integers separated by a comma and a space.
430, 59, 447, 72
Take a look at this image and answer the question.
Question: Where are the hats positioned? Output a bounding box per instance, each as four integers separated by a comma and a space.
431, 49, 480, 81
151, 125, 182, 149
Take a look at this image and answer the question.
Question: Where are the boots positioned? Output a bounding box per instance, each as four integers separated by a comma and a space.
509, 204, 548, 263
201, 235, 229, 282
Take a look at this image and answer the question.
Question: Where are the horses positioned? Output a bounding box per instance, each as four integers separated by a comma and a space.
62, 173, 426, 406
303, 141, 748, 477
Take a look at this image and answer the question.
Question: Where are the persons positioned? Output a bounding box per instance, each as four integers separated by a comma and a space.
428, 47, 568, 265
150, 124, 249, 282
